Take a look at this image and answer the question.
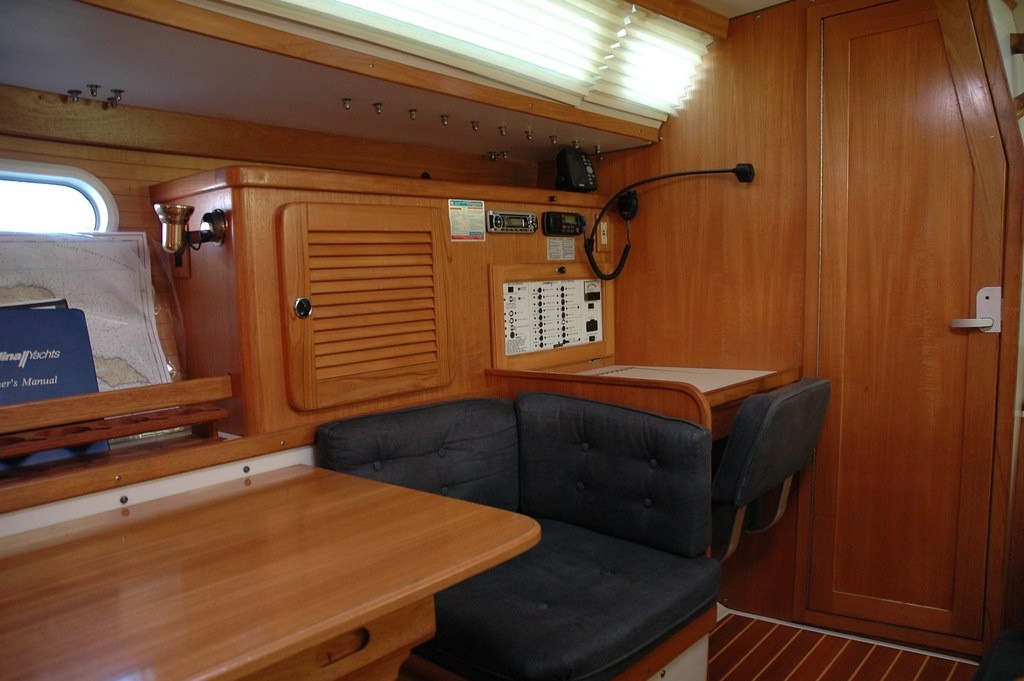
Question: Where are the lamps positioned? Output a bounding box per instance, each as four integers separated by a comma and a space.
154, 203, 227, 256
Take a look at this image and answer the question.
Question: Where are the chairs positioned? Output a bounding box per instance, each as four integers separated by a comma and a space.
712, 379, 831, 569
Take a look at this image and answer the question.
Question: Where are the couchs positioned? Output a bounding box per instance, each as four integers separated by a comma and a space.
315, 393, 723, 681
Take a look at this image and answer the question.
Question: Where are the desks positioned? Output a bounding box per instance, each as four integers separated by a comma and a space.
565, 361, 800, 446
0, 463, 542, 681
